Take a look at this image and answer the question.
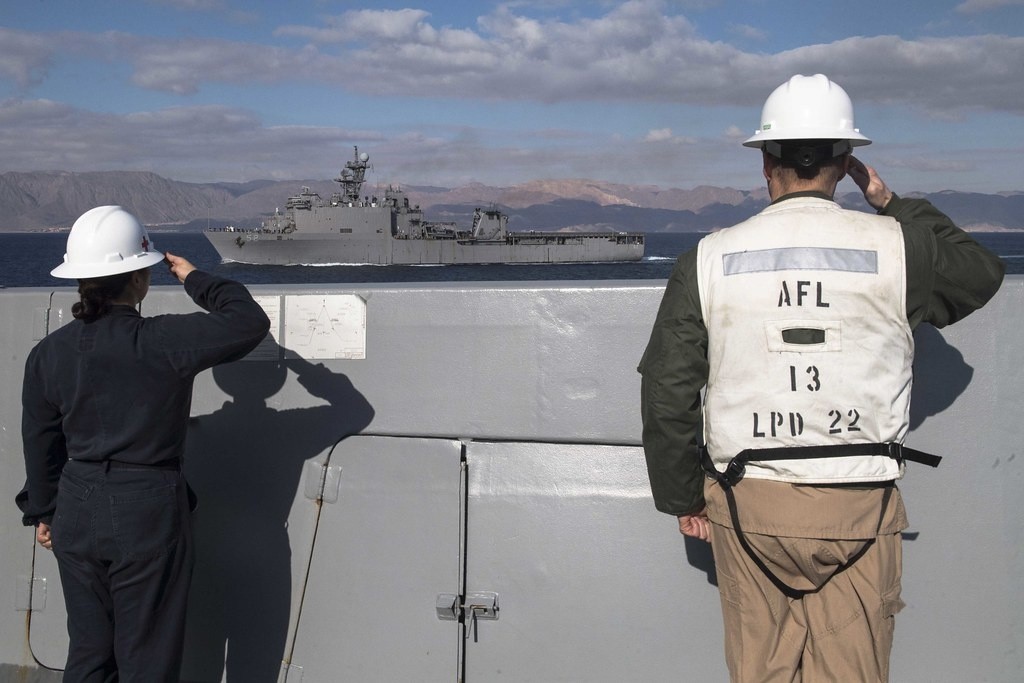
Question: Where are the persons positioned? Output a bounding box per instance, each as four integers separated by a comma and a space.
635, 74, 1006, 683
15, 206, 272, 683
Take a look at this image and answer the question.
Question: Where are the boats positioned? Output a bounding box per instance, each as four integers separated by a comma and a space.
202, 145, 645, 265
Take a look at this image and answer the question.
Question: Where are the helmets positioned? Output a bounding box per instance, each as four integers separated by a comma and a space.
742, 74, 872, 147
50, 206, 166, 278
